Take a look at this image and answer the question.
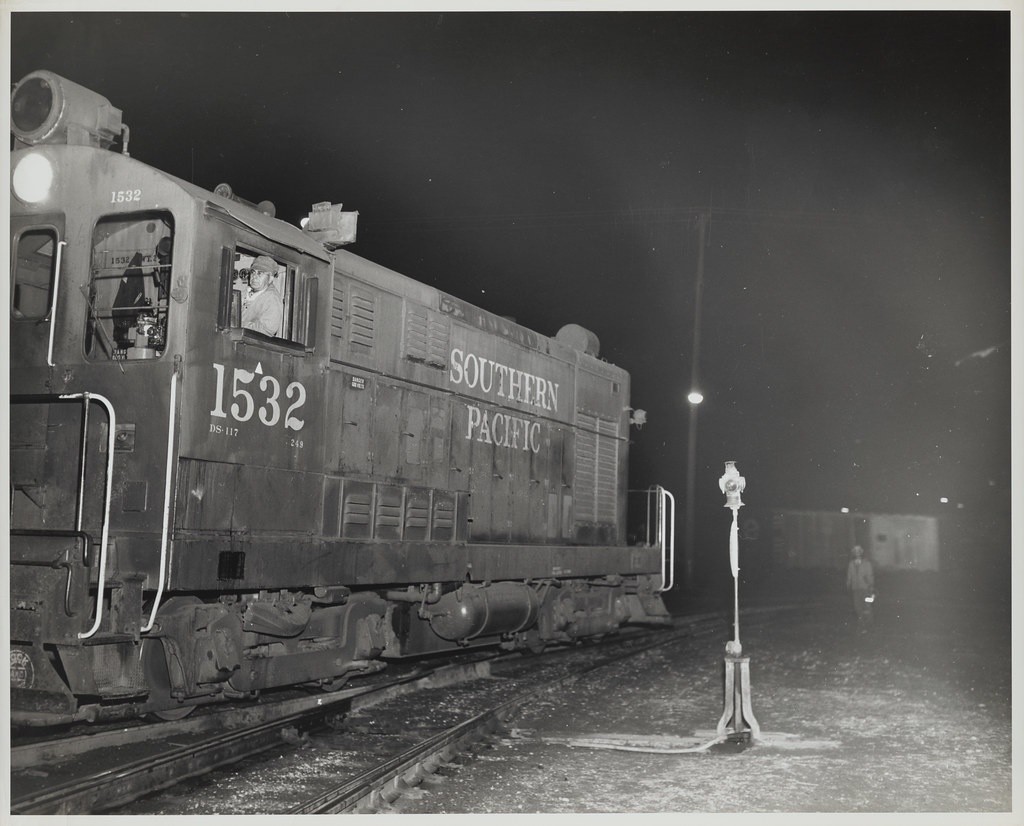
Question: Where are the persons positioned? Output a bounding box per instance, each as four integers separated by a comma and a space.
846, 546, 876, 627
240, 255, 282, 338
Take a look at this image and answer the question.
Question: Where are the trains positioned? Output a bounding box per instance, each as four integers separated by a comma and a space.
11, 68, 677, 715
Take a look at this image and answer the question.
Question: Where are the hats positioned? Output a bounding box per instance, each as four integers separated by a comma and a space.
851, 546, 863, 553
251, 256, 278, 275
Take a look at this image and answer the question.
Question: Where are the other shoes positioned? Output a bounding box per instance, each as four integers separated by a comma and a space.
856, 610, 870, 622
